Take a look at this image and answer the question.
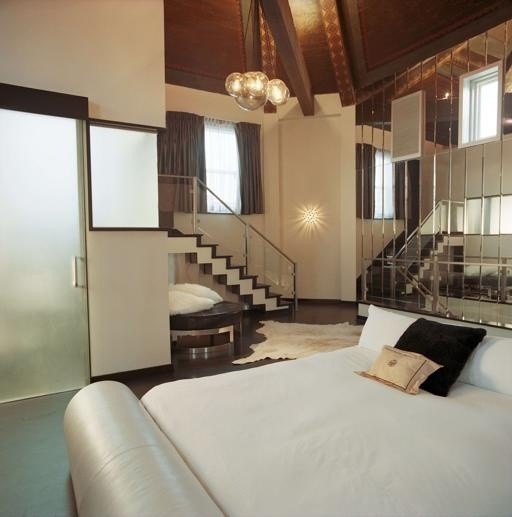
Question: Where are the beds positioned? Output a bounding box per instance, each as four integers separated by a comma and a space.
62, 305, 512, 517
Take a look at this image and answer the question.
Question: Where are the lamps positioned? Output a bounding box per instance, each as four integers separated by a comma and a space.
225, 70, 291, 116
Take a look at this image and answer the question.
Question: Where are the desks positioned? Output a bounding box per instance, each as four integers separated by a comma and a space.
170, 300, 243, 358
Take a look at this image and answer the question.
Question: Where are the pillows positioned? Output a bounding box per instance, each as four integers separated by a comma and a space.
169, 283, 224, 313
355, 318, 487, 399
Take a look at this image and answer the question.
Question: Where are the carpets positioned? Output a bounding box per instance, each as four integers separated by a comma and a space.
233, 320, 366, 365
